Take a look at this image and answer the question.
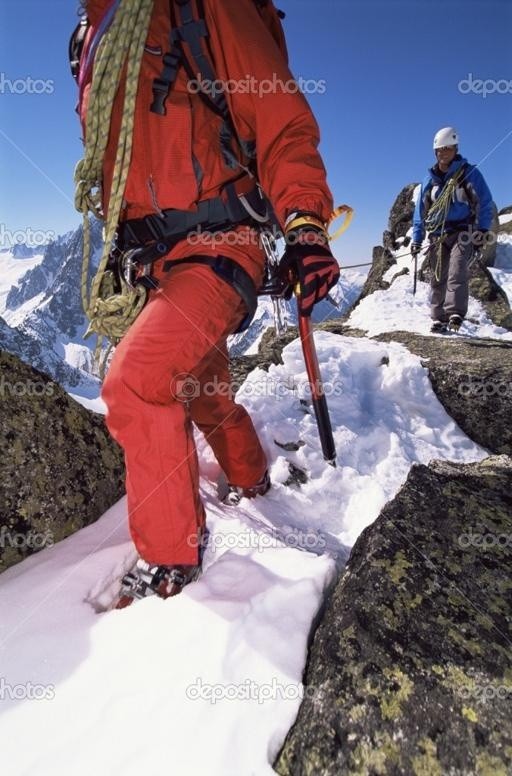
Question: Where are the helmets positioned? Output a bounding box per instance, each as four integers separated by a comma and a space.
433, 128, 458, 149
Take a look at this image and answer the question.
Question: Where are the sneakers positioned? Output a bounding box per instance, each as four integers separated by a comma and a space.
431, 322, 447, 332
222, 472, 270, 506
449, 316, 462, 329
107, 559, 200, 611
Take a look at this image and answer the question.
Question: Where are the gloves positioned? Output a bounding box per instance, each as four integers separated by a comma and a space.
411, 243, 420, 257
474, 229, 489, 249
278, 212, 340, 315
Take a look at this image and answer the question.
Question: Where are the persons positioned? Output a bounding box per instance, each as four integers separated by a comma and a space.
68, 1, 341, 615
409, 126, 493, 332
